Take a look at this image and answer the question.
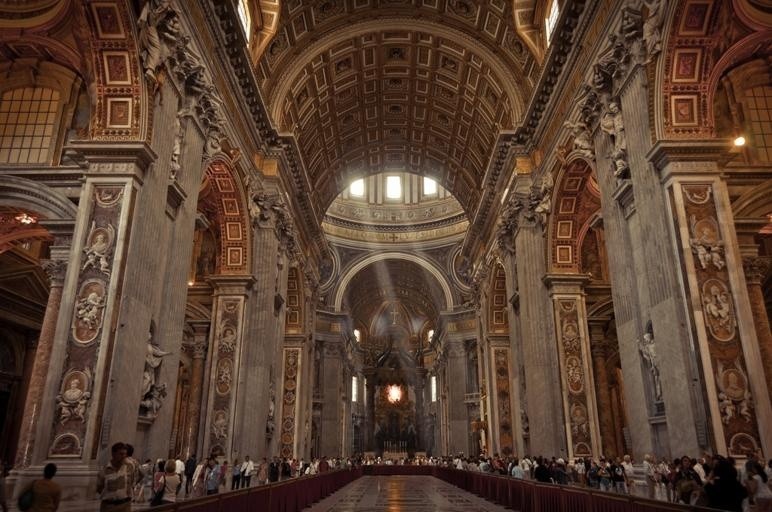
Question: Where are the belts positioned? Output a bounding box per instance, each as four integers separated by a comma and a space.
101, 496, 132, 505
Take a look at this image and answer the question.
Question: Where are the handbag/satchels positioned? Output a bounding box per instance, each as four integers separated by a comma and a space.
150, 475, 166, 506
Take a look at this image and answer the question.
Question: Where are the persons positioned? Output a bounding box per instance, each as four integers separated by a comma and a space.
89, 295, 106, 320
718, 392, 735, 425
135, 0, 233, 158
91, 248, 112, 278
346, 451, 446, 466
92, 436, 346, 512
63, 379, 82, 403
577, 1, 667, 114
710, 240, 727, 272
74, 390, 92, 424
80, 246, 97, 273
737, 386, 755, 424
563, 121, 595, 154
704, 296, 720, 320
140, 331, 177, 399
716, 293, 733, 321
689, 238, 711, 271
635, 331, 663, 401
75, 298, 91, 321
17, 463, 64, 512
86, 235, 108, 266
599, 102, 627, 178
56, 394, 73, 425
